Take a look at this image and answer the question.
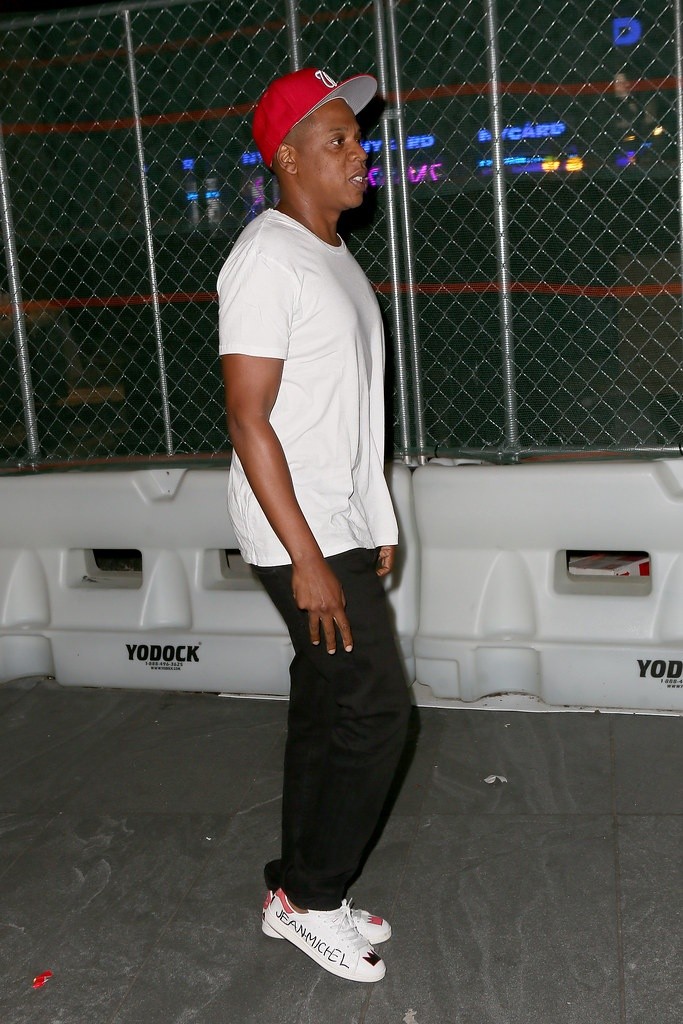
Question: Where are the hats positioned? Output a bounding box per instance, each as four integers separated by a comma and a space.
253, 66, 377, 168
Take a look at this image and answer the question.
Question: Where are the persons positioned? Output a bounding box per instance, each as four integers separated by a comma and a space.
215, 69, 415, 984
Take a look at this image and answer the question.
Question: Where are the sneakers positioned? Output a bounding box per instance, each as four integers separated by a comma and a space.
264, 888, 385, 983
262, 889, 391, 944
